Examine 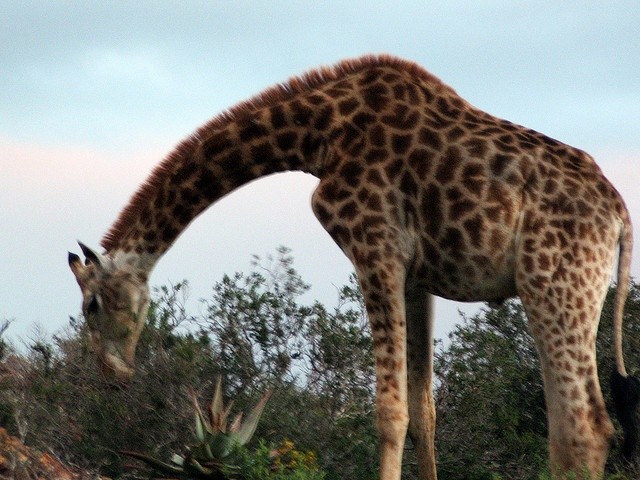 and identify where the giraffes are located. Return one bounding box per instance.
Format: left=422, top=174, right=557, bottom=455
left=67, top=54, right=640, bottom=480
